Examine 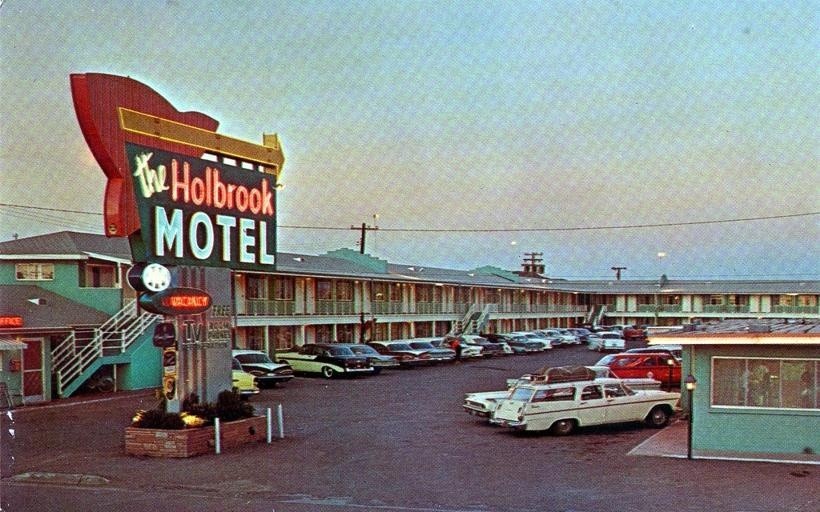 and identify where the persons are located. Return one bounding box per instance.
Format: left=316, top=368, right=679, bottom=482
left=448, top=339, right=462, bottom=363
